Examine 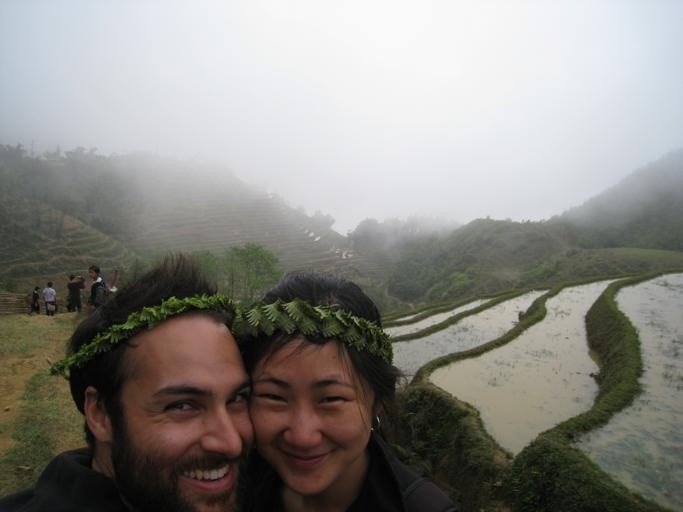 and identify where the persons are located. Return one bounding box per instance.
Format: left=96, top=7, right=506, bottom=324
left=88, top=264, right=107, bottom=311
left=234, top=270, right=455, bottom=511
left=67, top=274, right=89, bottom=312
left=0, top=247, right=255, bottom=511
left=42, top=282, right=56, bottom=316
left=27, top=286, right=42, bottom=315
